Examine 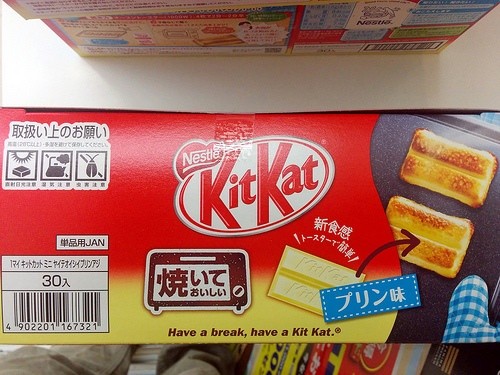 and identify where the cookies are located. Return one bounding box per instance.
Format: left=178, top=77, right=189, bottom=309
left=162, top=28, right=200, bottom=40
left=193, top=34, right=246, bottom=47
left=75, top=30, right=127, bottom=38
left=385, top=196, right=474, bottom=278
left=399, top=128, right=498, bottom=209
